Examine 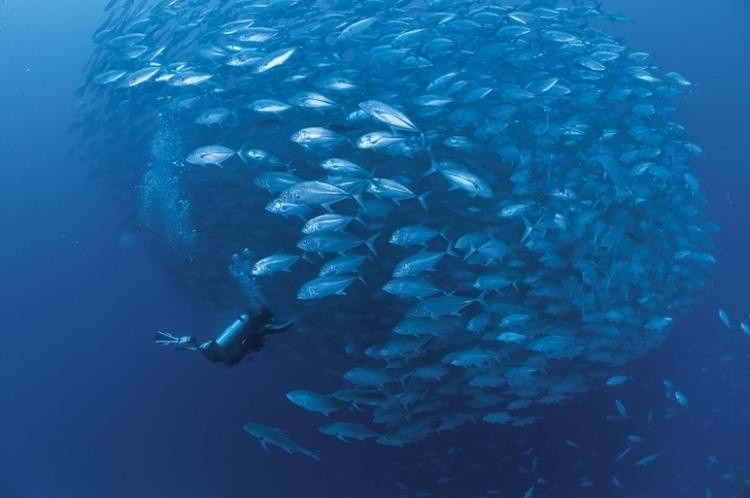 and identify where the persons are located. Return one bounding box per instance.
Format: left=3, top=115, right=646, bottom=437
left=153, top=306, right=294, bottom=368
